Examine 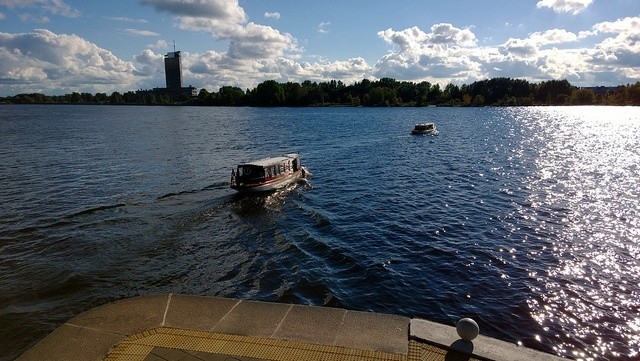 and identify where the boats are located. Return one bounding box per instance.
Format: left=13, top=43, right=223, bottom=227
left=411, top=122, right=436, bottom=135
left=229, top=153, right=311, bottom=195
